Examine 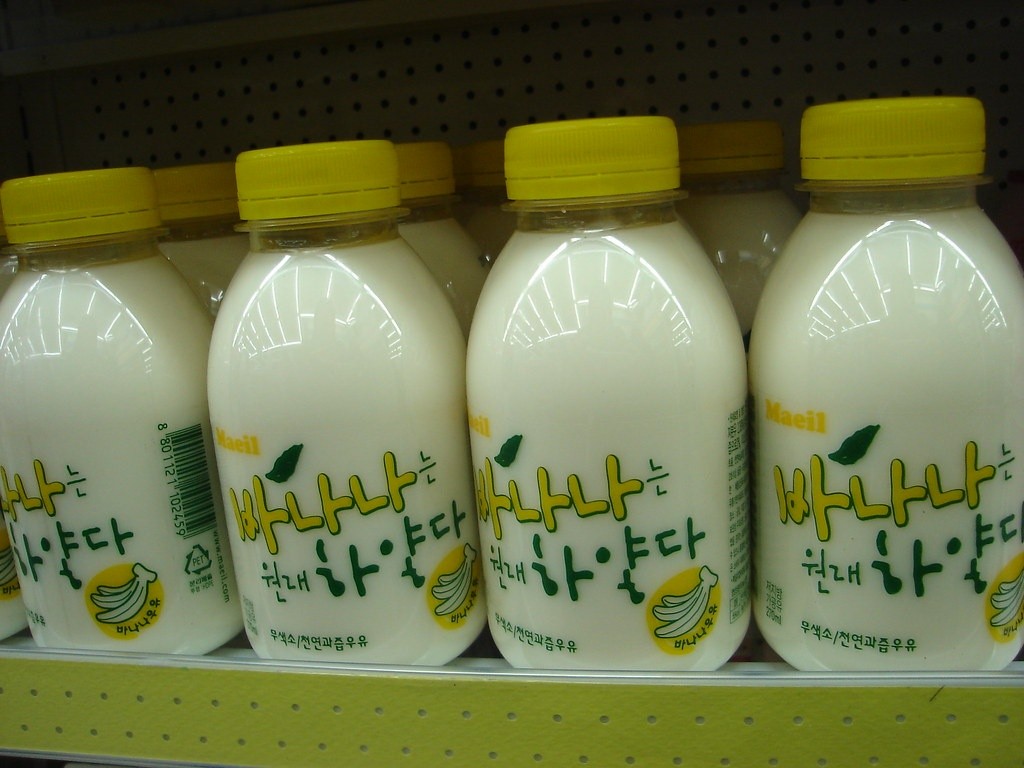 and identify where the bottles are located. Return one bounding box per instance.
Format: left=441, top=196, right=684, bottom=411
left=0, top=167, right=244, bottom=656
left=749, top=97, right=1024, bottom=673
left=146, top=159, right=248, bottom=320
left=390, top=140, right=496, bottom=334
left=673, top=116, right=803, bottom=347
left=467, top=114, right=749, bottom=675
left=460, top=129, right=509, bottom=262
left=203, top=138, right=485, bottom=666
